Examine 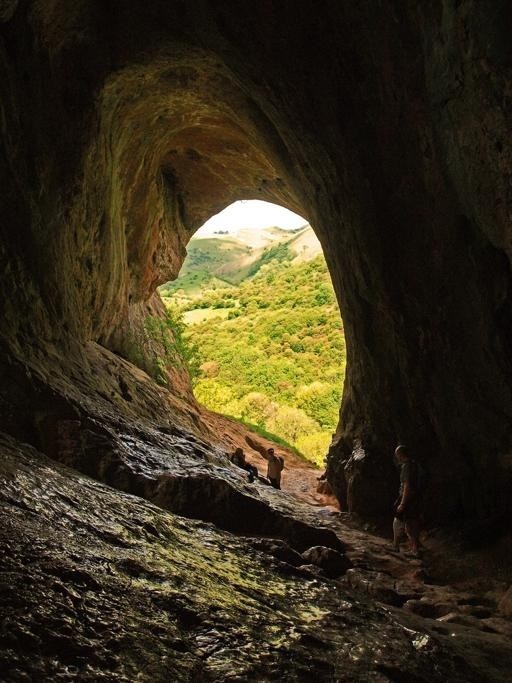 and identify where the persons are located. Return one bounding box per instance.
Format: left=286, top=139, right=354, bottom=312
left=382, top=445, right=421, bottom=558
left=233, top=448, right=258, bottom=479
left=259, top=446, right=281, bottom=489
